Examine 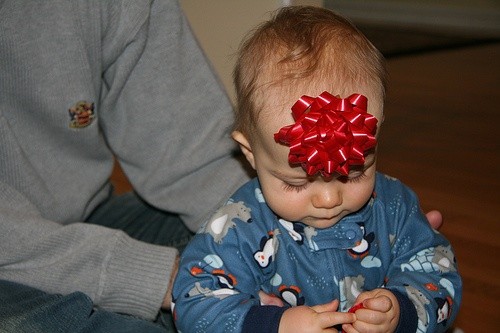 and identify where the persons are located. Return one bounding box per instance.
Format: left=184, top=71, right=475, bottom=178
left=170, top=6, right=464, bottom=333
left=0, top=1, right=445, bottom=333
